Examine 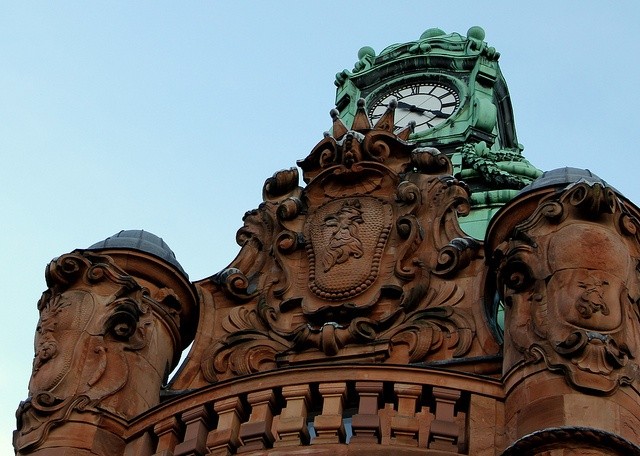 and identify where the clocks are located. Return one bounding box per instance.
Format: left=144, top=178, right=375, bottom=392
left=365, top=80, right=460, bottom=136
